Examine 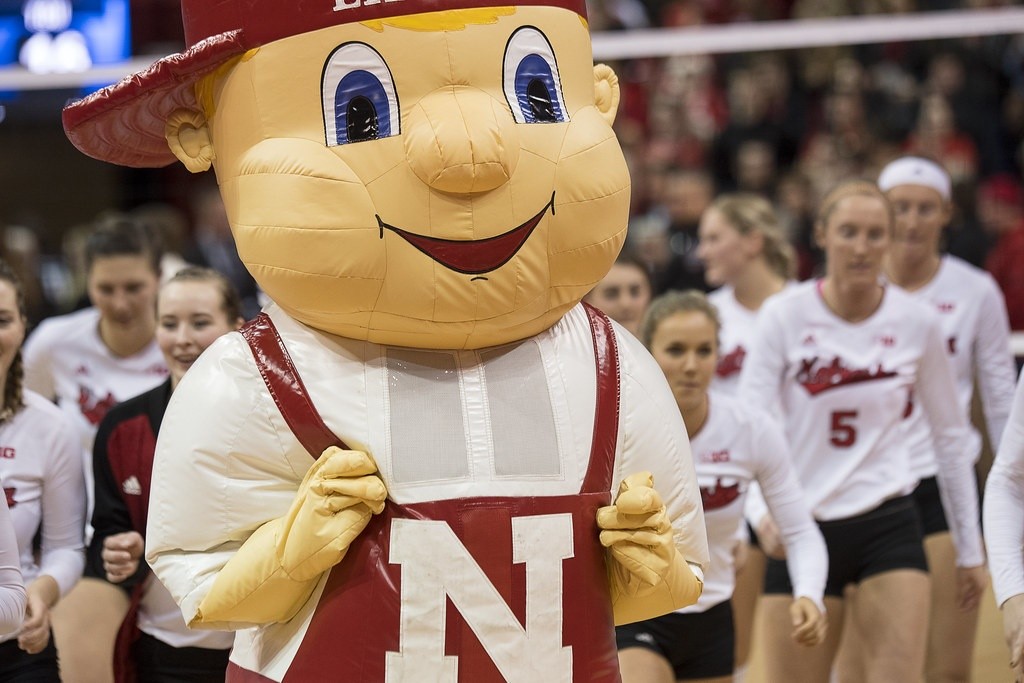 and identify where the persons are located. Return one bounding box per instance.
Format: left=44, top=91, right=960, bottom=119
left=0, top=1, right=1024, bottom=683
left=60, top=1, right=710, bottom=683
